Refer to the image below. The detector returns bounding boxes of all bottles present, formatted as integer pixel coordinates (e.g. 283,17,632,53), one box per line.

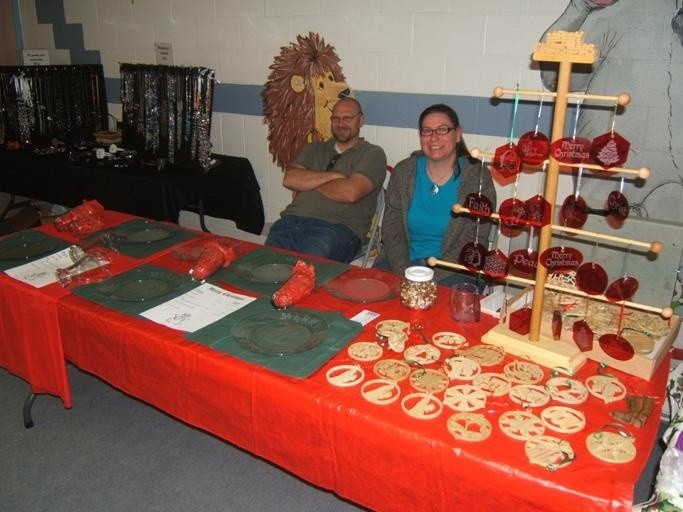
402,266,438,310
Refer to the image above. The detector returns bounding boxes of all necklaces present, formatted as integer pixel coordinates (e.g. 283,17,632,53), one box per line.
428,158,456,195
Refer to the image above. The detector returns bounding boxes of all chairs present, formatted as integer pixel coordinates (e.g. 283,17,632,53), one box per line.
351,164,502,300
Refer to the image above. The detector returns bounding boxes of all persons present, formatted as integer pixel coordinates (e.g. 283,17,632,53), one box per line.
371,104,496,295
264,97,386,264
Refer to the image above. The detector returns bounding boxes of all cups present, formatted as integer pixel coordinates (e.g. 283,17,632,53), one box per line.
54,204,110,288
190,247,226,284
273,272,310,306
451,285,480,320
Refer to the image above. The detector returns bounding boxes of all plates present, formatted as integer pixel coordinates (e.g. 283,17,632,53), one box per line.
105,270,183,302
1,237,55,262
327,270,400,300
234,253,300,285
234,311,329,356
114,222,177,244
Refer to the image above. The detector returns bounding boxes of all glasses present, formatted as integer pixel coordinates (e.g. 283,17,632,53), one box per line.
329,111,359,123
419,125,457,135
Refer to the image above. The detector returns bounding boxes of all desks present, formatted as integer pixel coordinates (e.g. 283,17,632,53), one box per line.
3,148,266,238
1,191,206,431
56,230,682,510
